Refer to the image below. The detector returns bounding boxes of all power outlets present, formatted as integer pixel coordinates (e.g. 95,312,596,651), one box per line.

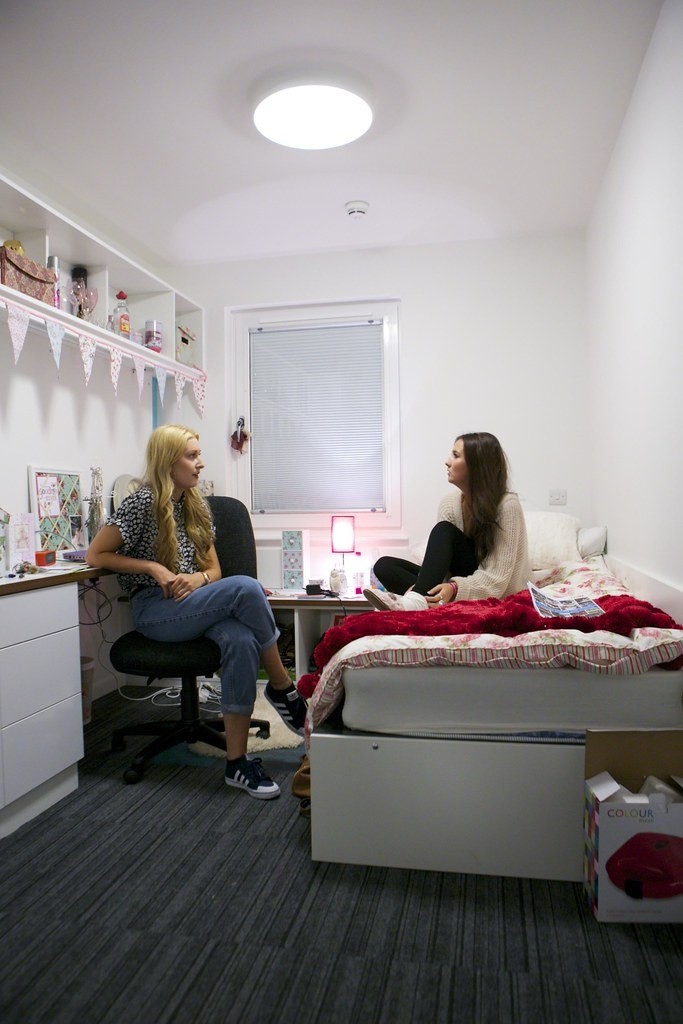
115,593,131,607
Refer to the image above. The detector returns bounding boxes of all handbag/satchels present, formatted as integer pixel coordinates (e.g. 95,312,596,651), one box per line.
291,754,311,815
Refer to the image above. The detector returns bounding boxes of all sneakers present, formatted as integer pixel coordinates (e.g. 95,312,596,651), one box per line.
226,755,281,799
264,679,310,737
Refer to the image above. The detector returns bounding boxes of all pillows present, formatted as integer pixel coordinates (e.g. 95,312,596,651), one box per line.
520,511,583,569
576,524,607,557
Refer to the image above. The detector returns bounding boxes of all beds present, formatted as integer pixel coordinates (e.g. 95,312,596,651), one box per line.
310,553,683,885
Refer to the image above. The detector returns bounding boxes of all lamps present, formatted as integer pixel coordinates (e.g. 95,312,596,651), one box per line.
251,85,376,150
330,515,355,566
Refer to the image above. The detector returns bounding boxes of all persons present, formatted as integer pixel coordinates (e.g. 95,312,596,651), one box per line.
86,424,309,799
362,431,532,612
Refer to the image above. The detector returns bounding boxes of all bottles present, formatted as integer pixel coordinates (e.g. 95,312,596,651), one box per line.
349,551,368,598
113,291,131,340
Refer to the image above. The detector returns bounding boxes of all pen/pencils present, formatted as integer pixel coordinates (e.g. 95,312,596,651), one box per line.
3,573,16,578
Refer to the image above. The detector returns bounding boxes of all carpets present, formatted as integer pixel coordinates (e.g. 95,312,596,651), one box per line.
187,688,304,758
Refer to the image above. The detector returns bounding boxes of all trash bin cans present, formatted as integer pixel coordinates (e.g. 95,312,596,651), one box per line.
81,656,95,725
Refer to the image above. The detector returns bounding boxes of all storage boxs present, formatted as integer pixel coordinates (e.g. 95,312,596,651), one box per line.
581,760,683,925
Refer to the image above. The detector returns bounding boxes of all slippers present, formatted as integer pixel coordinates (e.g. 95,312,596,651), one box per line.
363,588,405,612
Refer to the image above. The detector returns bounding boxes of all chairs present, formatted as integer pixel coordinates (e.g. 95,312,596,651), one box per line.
109,495,271,785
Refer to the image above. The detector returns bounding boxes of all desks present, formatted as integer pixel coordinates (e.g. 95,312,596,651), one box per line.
263,590,376,681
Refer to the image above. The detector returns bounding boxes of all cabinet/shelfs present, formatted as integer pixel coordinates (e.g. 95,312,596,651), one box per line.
0,559,112,841
0,173,205,380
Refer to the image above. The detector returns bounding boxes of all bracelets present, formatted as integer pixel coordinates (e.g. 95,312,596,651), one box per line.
450,582,457,599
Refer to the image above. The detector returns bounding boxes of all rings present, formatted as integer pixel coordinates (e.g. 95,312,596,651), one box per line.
438,594,443,600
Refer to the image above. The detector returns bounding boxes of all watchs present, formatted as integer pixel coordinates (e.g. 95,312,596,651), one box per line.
200,571,211,585
